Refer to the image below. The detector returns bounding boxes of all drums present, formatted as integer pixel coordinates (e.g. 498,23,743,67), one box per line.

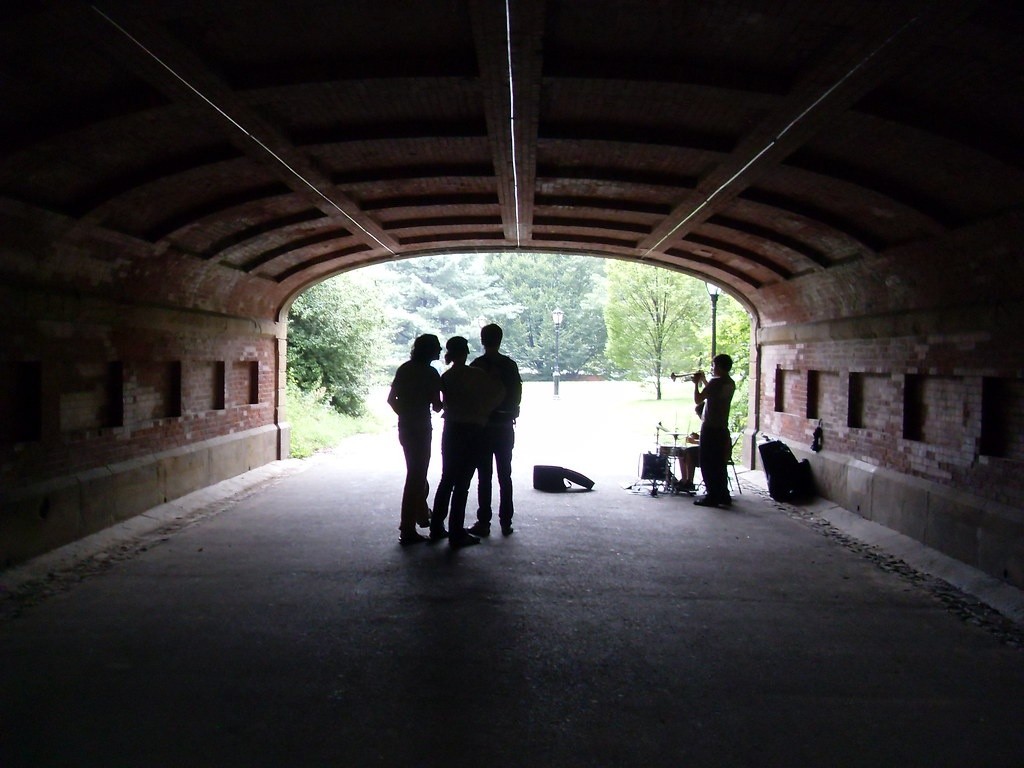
658,445,686,457
639,454,669,480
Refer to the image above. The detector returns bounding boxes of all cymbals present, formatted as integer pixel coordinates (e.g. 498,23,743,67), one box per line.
653,422,670,432
667,433,689,436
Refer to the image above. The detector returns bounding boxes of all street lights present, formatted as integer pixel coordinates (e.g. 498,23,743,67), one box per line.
552,307,564,395
704,280,721,374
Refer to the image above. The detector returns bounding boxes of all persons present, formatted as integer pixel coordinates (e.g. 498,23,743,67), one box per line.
693,354,736,505
387,324,522,548
675,403,732,490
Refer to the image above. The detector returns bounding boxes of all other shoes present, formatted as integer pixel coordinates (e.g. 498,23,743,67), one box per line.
433,528,450,540
674,479,695,490
420,509,433,529
694,498,718,506
502,524,514,536
466,522,490,536
450,533,480,548
399,533,431,546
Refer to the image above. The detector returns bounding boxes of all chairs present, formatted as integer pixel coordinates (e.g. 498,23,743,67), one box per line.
697,432,742,494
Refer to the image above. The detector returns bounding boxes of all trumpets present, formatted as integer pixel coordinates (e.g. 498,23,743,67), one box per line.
671,370,714,388
